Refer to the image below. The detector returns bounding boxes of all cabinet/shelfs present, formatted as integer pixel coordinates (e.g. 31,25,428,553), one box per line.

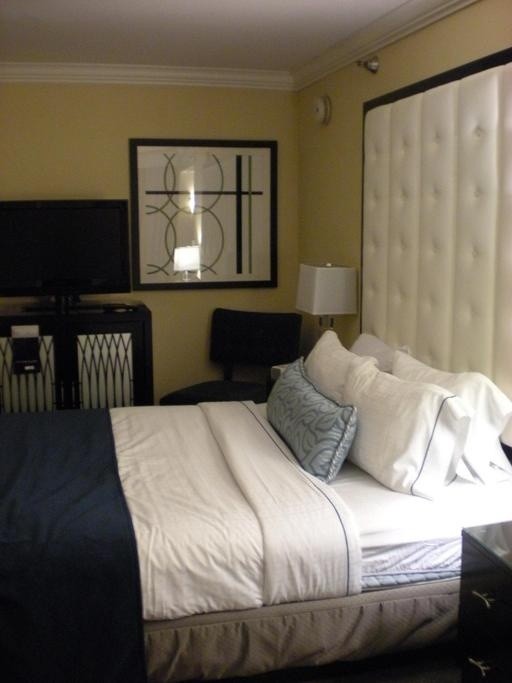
0,300,154,414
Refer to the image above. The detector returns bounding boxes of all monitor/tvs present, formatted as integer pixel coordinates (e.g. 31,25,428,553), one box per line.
0,198,131,312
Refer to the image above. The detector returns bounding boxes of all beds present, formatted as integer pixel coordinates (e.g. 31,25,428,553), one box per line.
0,329,512,683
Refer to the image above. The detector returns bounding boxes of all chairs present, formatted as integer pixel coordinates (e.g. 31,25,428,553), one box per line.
160,307,303,405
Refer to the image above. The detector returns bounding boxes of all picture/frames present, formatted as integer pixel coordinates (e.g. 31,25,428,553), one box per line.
129,139,279,291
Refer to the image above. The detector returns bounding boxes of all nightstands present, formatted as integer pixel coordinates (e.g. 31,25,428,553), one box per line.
459,520,512,683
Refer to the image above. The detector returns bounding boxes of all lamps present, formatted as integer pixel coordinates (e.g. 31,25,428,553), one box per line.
295,261,358,340
172,245,200,281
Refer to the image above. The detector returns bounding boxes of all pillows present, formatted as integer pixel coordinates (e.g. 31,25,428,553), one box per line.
392,350,512,486
266,357,359,483
348,332,409,373
303,328,379,409
340,355,472,501
